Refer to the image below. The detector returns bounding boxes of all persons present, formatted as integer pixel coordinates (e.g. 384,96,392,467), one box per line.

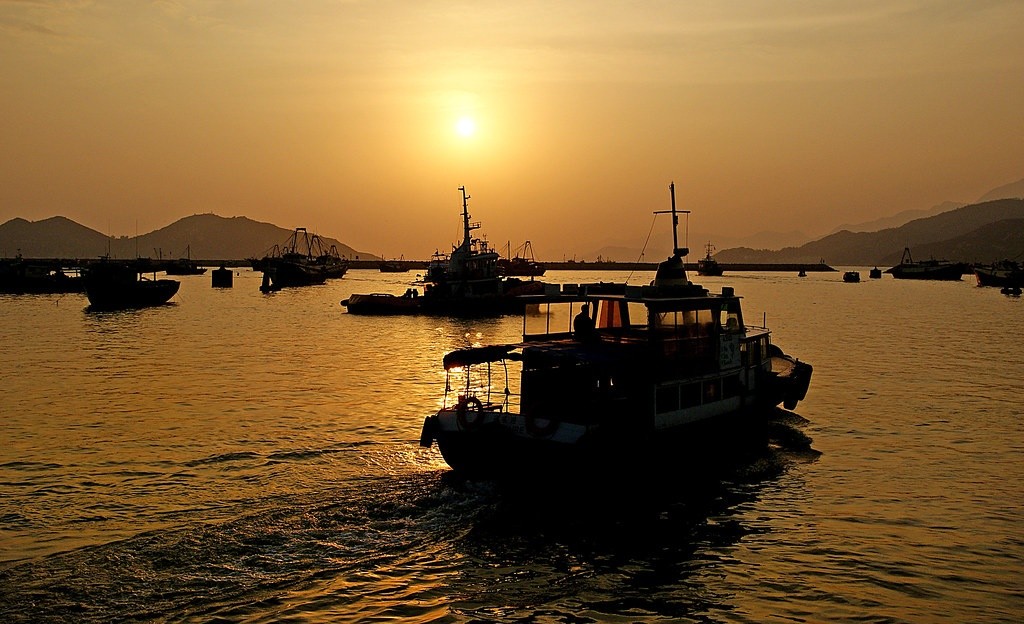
572,303,602,342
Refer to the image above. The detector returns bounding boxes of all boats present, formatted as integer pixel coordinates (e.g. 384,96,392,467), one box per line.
0,252,82,297
696,240,725,276
247,227,351,297
377,254,412,273
798,268,807,277
843,270,860,283
339,186,546,319
83,253,180,314
418,180,814,522
975,260,1024,289
886,246,972,282
138,244,207,275
